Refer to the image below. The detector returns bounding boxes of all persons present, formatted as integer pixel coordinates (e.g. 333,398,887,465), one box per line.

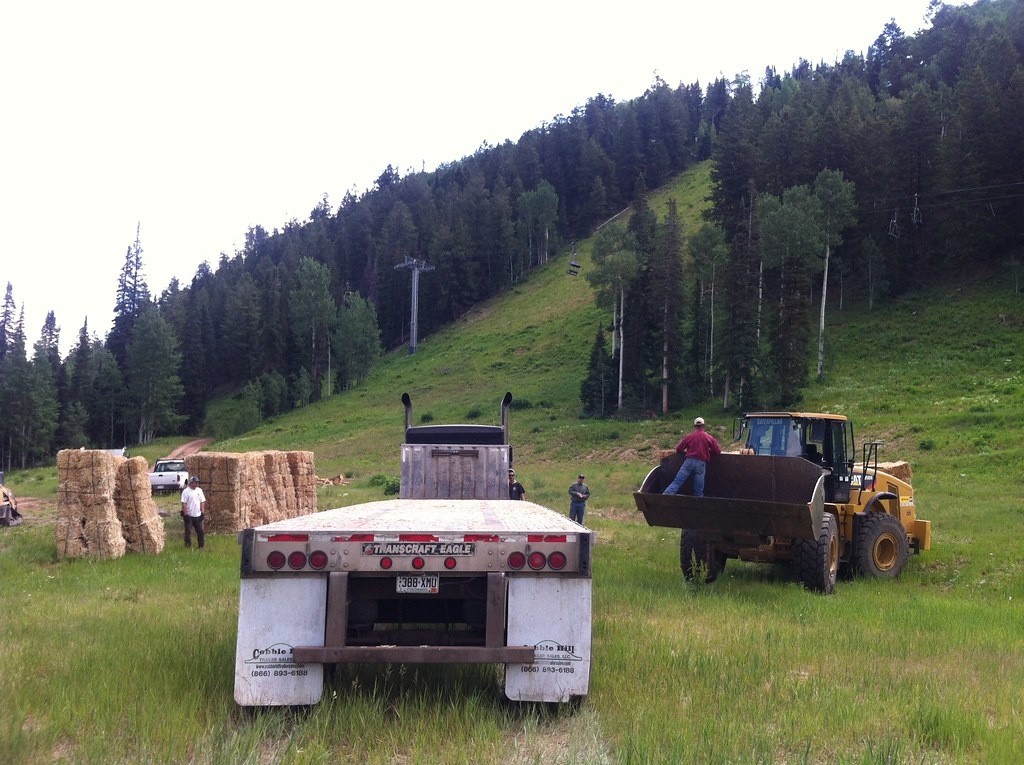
568,474,590,525
663,417,721,497
509,470,526,501
181,477,207,547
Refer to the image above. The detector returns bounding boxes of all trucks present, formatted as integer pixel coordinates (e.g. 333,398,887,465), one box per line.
235,392,597,718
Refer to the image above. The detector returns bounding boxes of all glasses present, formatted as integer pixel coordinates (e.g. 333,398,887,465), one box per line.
509,475,515,477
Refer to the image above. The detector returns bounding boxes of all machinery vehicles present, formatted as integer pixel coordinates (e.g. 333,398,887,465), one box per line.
632,409,931,597
0,471,23,528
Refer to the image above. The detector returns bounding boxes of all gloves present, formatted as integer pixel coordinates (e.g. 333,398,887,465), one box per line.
184,515,188,520
199,513,204,521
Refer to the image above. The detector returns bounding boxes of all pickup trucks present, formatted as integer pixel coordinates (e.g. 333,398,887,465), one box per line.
147,458,190,495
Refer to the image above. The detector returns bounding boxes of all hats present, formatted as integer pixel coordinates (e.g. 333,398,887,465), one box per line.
509,469,516,473
694,417,704,427
190,477,200,483
579,475,584,479
